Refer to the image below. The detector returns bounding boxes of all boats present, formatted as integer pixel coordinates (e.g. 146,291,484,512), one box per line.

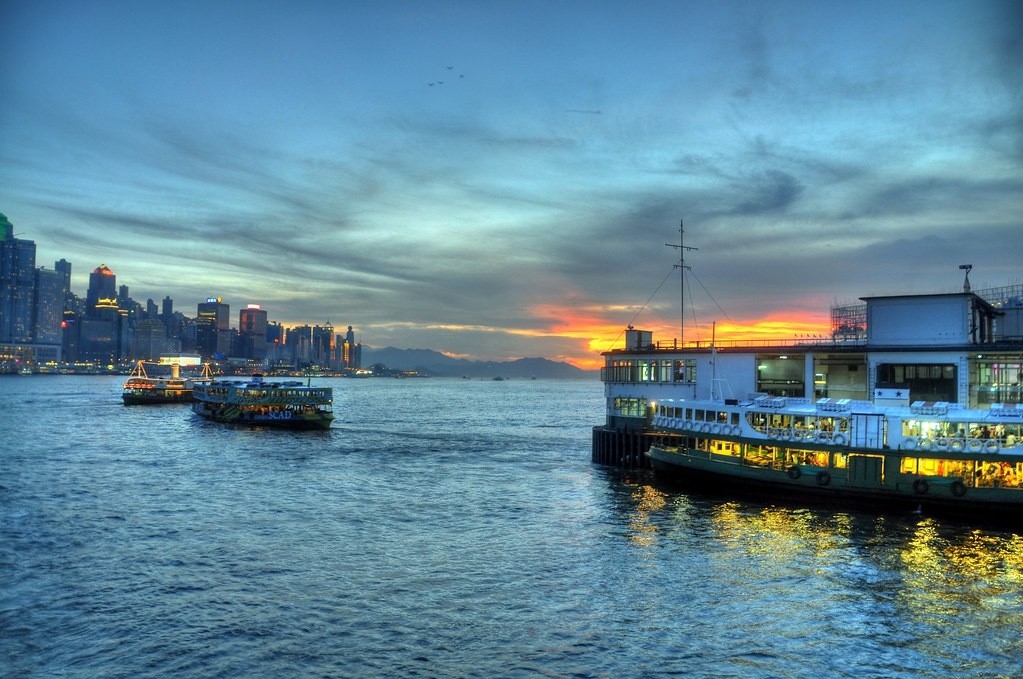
646,320,1023,510
191,362,336,429
121,359,215,405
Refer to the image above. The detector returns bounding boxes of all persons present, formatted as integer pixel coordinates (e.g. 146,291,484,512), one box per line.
980,427,990,439
1007,432,1016,447
795,417,829,437
774,419,790,435
956,429,964,438
961,462,968,476
938,461,943,476
976,462,1016,488
791,451,814,466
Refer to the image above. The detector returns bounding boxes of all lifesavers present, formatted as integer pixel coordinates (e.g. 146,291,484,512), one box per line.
913,479,929,494
950,481,966,497
655,416,742,437
903,437,1000,455
769,427,846,444
816,471,831,485
788,466,801,479
